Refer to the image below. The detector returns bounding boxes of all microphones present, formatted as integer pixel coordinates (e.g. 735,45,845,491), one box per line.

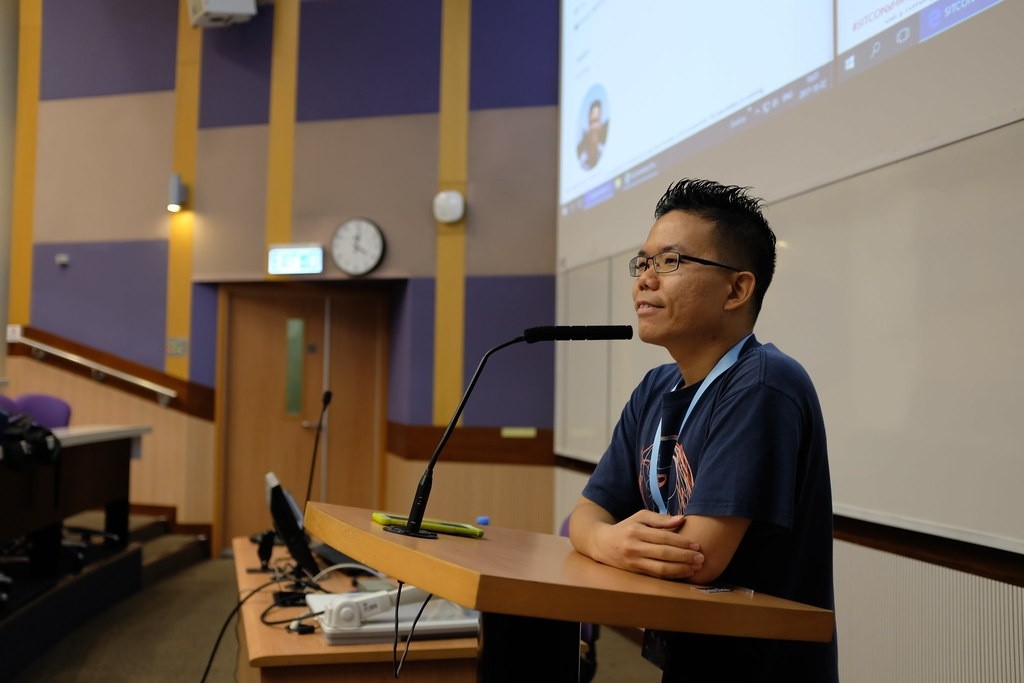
303,390,332,514
405,324,633,532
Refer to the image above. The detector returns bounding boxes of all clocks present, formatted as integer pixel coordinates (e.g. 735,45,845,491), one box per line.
331,218,386,276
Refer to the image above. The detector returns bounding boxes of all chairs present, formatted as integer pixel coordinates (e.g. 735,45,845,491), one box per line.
0,396,72,429
559,512,600,683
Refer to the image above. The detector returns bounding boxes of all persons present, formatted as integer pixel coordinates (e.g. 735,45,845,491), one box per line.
569,179,839,683
578,101,605,171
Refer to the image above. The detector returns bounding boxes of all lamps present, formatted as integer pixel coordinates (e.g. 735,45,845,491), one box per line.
166,172,189,214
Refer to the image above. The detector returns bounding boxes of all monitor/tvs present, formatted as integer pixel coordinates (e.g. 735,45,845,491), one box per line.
266,473,320,576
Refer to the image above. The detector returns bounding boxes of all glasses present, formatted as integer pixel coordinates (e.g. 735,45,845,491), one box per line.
628,251,743,277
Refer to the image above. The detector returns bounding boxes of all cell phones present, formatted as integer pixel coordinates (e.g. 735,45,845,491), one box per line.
371,513,484,537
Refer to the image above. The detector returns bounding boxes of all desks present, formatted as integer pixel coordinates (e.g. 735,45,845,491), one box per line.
0,423,154,611
231,534,477,683
